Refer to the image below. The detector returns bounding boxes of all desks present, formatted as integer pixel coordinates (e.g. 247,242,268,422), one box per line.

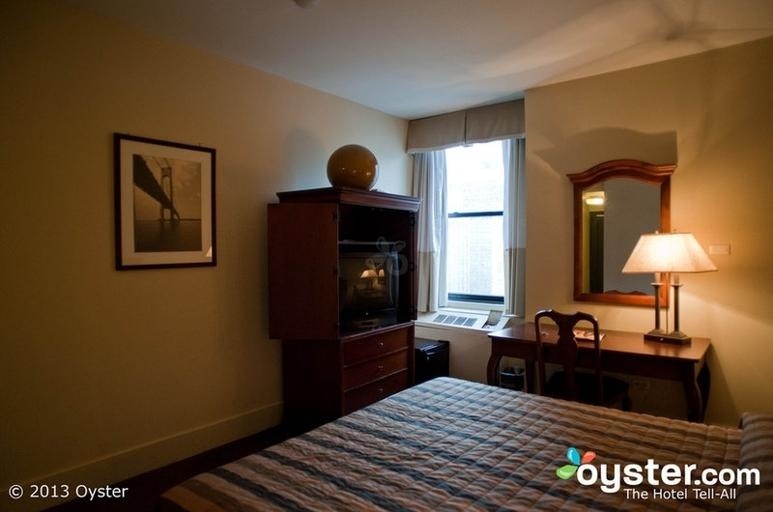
484,320,714,422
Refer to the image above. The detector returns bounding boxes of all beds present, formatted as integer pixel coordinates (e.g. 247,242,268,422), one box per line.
152,376,741,512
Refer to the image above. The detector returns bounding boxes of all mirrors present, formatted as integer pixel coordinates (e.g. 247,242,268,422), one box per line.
563,159,678,308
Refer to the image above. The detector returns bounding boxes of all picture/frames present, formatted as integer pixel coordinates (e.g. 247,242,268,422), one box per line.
113,131,216,274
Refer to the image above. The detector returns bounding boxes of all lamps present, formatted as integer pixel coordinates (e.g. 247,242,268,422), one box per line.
622,230,718,346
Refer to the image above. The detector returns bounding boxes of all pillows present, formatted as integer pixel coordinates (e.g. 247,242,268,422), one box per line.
733,409,771,512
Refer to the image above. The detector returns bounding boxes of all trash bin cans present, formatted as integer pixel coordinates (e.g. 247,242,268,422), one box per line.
498,366,525,392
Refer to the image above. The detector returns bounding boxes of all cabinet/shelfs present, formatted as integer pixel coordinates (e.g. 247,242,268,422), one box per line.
263,187,450,441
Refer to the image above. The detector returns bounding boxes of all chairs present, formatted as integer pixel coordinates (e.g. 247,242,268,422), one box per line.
534,308,604,409
